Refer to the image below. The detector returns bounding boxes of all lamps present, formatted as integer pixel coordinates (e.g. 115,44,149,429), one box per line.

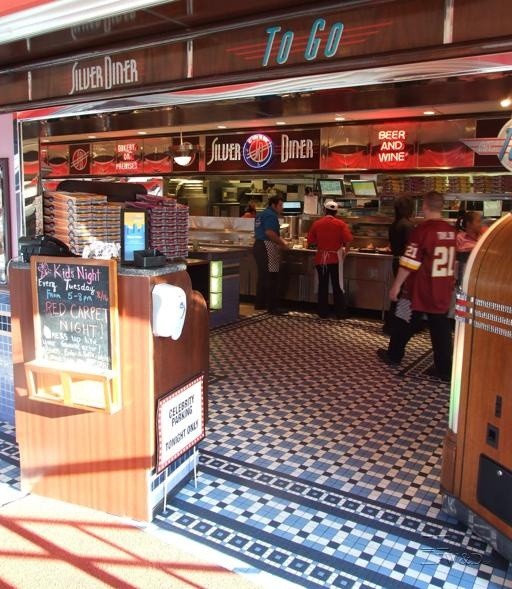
168,132,194,166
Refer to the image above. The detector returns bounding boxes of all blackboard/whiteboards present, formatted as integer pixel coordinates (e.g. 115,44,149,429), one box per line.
30,255,121,371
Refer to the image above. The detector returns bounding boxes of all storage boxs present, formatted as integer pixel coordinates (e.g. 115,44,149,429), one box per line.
376,172,510,200
41,187,192,265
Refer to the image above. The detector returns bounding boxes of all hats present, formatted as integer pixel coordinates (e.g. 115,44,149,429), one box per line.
323,201,338,211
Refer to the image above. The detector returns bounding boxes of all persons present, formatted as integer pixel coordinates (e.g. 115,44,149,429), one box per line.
383,195,415,335
306,200,353,319
455,209,481,252
377,191,456,380
252,195,289,316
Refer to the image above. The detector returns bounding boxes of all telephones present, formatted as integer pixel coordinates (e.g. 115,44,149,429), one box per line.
19,235,70,263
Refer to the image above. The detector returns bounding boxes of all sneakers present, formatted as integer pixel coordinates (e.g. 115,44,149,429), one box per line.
332,313,353,320
254,303,289,316
376,347,402,366
427,366,451,381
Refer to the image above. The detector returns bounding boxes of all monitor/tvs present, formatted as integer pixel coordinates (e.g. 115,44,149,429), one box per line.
350,180,378,197
483,200,502,218
317,179,345,197
